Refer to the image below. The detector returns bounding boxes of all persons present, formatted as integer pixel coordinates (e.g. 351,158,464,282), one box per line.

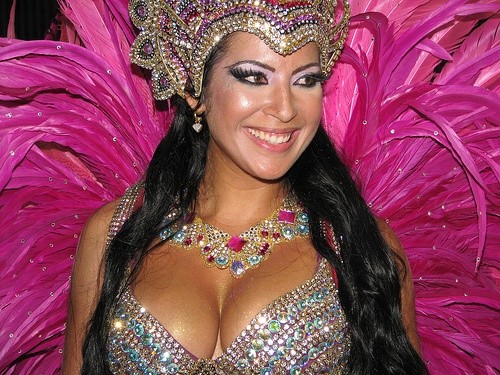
50,0,429,375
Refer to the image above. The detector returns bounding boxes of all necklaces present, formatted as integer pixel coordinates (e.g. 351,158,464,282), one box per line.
157,190,311,279
